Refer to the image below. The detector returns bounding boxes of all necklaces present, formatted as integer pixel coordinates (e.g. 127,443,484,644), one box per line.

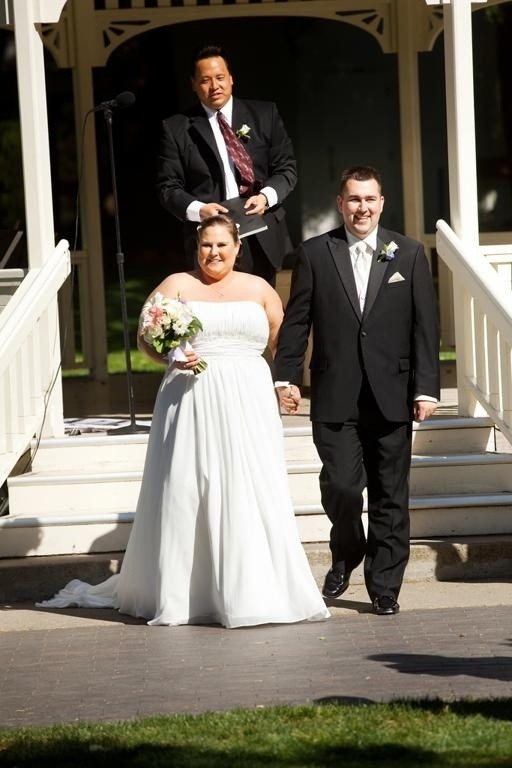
214,285,226,297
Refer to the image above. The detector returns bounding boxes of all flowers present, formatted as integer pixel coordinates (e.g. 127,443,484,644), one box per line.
137,292,212,375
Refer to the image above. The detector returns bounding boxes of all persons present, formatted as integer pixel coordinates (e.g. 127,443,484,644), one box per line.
154,44,300,287
272,160,449,614
132,213,306,633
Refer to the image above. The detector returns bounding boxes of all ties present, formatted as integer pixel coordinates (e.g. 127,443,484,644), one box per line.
351,242,369,316
214,109,256,195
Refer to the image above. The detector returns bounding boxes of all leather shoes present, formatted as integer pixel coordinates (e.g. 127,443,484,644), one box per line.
372,594,400,615
322,564,351,599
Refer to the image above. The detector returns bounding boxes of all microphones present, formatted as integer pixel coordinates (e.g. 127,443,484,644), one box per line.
89,91,136,112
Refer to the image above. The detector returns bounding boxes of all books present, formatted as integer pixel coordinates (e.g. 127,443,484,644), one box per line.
219,195,268,240
